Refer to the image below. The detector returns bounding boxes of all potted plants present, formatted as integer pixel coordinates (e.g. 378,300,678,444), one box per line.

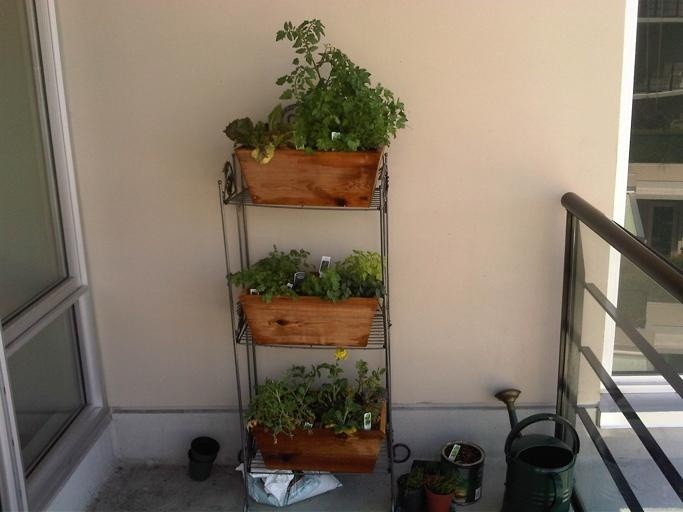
229,17,406,206
236,249,388,347
399,465,430,512
425,469,462,512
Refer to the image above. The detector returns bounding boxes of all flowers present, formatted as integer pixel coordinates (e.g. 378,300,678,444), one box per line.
248,351,384,434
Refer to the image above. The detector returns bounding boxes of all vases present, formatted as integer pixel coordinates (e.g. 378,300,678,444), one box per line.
188,437,220,480
253,425,384,472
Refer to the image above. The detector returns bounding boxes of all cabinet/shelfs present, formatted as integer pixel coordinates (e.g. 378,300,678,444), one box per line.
218,149,407,512
